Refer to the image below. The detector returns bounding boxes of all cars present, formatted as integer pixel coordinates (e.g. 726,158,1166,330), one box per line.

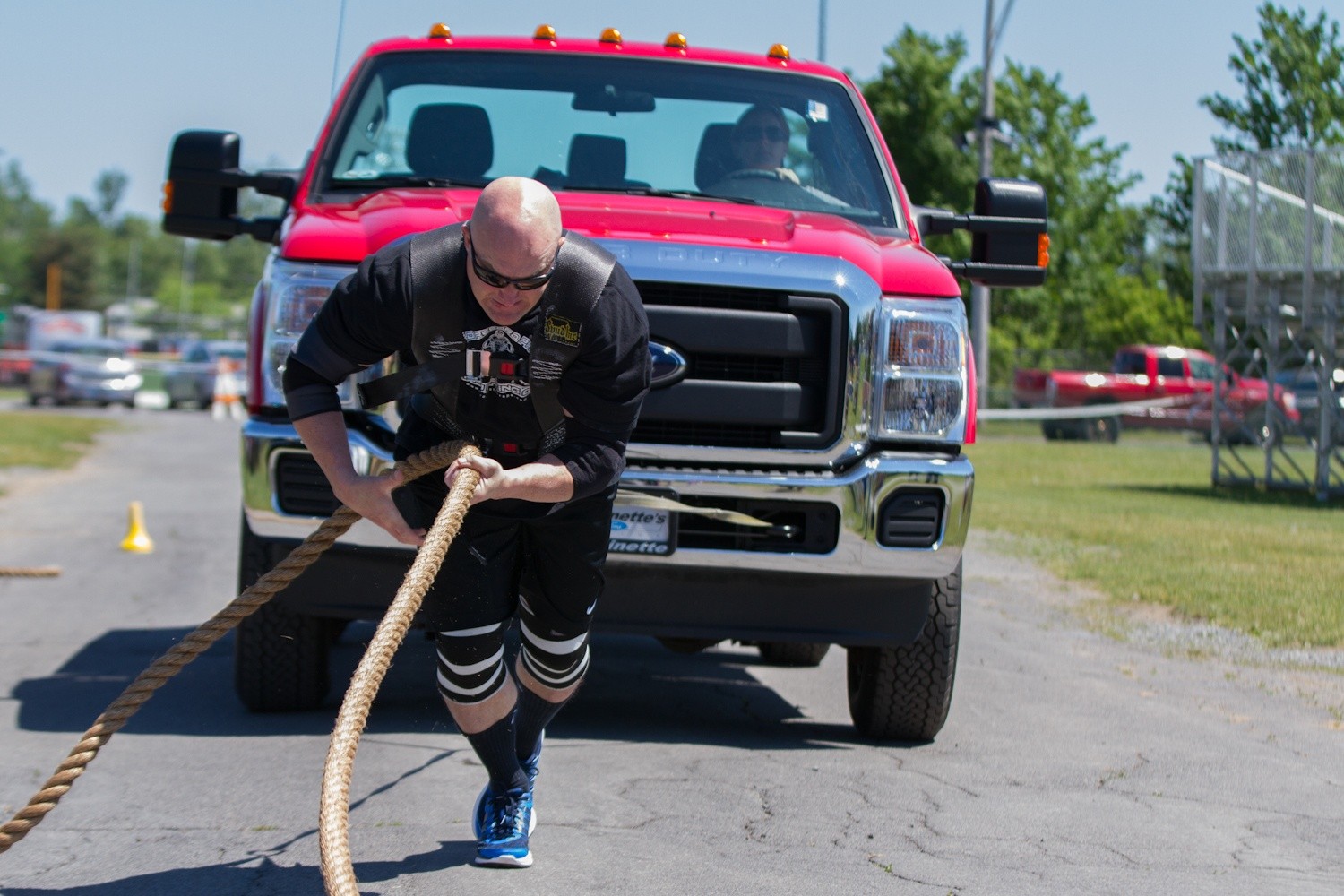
0,305,248,415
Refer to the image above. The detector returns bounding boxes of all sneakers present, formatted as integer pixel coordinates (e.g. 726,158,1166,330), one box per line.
472,720,541,835
475,783,539,868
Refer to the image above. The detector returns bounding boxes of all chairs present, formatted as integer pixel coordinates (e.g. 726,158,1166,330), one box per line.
378,104,496,182
693,123,741,191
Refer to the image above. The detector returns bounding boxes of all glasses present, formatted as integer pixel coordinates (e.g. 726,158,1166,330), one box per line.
743,125,785,142
469,226,559,291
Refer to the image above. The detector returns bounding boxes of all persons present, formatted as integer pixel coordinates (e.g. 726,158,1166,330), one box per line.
725,95,853,207
283,175,652,869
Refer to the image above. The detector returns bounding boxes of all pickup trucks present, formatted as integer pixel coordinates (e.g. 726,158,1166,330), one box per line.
1014,347,1302,447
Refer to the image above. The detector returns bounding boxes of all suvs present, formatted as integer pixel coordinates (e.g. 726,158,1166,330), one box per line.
157,21,1054,753
